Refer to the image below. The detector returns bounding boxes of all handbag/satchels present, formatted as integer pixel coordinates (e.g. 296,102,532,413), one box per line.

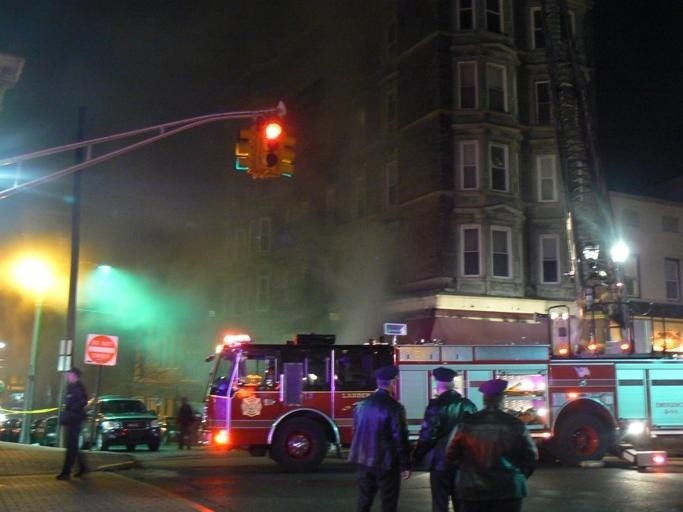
409,444,439,471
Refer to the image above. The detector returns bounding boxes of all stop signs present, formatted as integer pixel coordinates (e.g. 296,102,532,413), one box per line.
83,332,119,367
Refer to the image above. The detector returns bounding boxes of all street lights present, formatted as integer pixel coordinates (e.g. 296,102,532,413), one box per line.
15,254,114,446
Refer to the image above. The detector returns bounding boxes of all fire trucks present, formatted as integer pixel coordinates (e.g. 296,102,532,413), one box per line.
195,1,681,475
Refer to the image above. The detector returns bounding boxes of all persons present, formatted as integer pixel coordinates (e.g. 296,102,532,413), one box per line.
176,396,192,450
56,368,87,480
349,365,413,512
413,367,478,511
444,378,540,512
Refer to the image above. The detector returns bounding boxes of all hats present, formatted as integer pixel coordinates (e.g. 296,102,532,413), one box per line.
479,379,507,395
375,364,398,380
67,367,82,375
433,366,457,382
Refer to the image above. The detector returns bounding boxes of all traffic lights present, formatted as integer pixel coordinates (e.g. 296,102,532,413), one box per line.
273,134,300,178
229,124,257,174
255,115,286,171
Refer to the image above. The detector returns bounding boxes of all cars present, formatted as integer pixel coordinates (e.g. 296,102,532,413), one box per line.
156,412,210,448
0,415,59,448
77,394,162,453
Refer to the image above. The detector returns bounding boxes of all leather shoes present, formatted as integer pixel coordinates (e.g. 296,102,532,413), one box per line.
57,472,69,480
